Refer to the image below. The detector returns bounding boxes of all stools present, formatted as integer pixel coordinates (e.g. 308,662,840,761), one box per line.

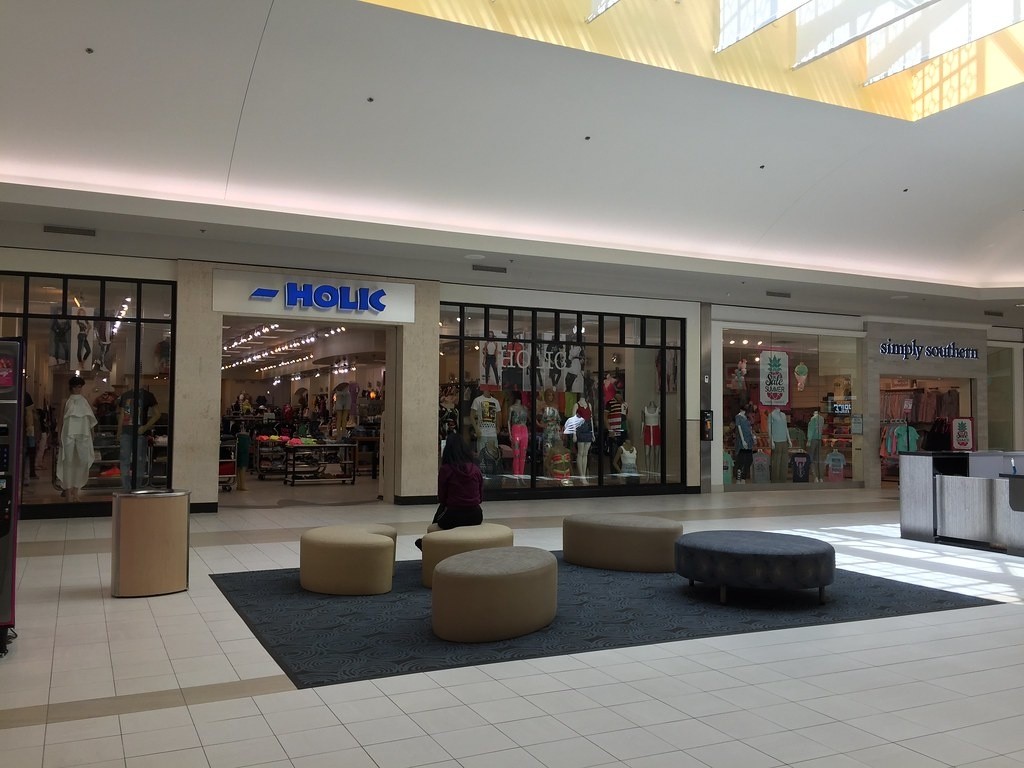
300,523,397,594
674,530,835,605
562,513,683,572
420,522,513,587
430,546,557,641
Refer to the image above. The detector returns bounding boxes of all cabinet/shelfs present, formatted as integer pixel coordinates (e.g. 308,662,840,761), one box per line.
218,413,380,492
51,426,168,490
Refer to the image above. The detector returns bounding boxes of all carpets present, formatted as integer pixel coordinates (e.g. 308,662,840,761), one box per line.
209,551,1006,691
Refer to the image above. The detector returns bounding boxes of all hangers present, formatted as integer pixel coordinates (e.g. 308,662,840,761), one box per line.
882,419,909,425
884,387,958,396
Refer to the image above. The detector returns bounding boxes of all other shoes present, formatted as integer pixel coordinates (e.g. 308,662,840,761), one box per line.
99,364,110,372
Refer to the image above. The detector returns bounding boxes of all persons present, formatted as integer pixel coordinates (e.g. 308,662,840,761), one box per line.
602,373,617,412
546,334,562,392
237,431,249,490
116,374,160,495
805,411,825,483
94,313,114,373
470,390,503,454
415,428,483,568
767,408,793,484
505,400,528,475
481,331,500,389
440,399,458,439
734,409,757,484
56,376,98,503
54,307,91,367
571,396,595,486
536,388,564,476
604,394,628,479
476,440,503,473
503,334,523,391
528,336,544,389
334,388,351,442
613,439,638,481
154,335,170,374
565,337,584,394
640,402,662,483
